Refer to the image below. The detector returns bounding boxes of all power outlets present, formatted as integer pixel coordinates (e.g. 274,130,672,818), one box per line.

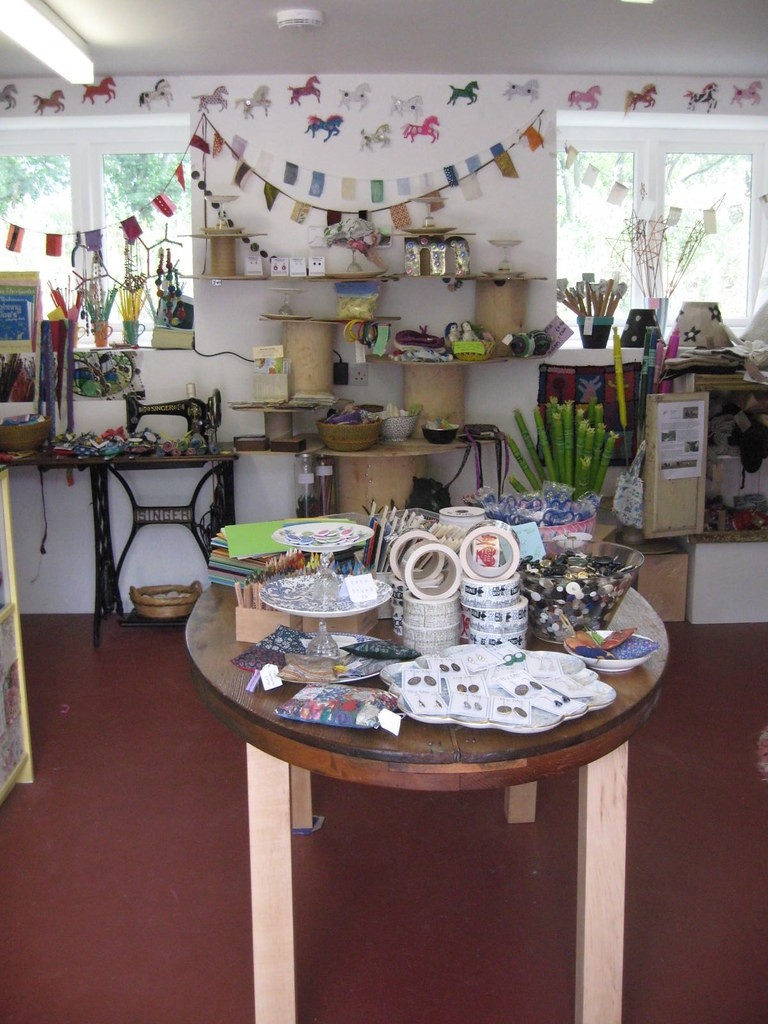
350,364,368,386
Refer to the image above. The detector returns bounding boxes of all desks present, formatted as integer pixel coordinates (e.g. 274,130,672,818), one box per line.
185,581,667,1024
314,441,494,516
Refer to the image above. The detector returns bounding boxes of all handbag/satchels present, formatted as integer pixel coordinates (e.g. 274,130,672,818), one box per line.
610,439,645,529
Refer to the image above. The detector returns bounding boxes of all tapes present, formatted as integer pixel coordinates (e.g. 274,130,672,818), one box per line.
461,520,520,582
401,620,462,655
467,624,527,650
468,595,530,634
389,572,445,608
392,606,403,636
402,589,462,627
460,571,521,609
400,540,462,601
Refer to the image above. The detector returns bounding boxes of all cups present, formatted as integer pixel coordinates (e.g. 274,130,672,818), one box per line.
94,321,113,347
123,320,145,345
74,322,86,347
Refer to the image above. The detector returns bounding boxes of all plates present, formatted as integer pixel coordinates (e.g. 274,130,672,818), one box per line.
258,575,393,617
323,272,386,279
261,314,313,320
271,523,374,552
482,271,527,277
268,631,396,683
489,240,523,246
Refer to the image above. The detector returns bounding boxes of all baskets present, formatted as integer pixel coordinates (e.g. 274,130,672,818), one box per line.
130,580,203,619
0,414,52,451
317,403,385,450
452,341,498,361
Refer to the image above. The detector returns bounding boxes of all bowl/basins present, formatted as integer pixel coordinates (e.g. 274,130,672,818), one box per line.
381,416,418,442
563,630,654,672
515,539,645,645
314,417,383,451
421,424,460,444
0,414,53,452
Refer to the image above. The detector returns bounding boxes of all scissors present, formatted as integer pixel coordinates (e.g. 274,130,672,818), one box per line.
483,490,600,526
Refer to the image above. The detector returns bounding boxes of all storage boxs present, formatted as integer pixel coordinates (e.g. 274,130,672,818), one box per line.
382,508,439,519
322,512,370,529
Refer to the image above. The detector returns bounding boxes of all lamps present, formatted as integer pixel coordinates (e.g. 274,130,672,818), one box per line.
0,0,94,85
276,10,324,29
